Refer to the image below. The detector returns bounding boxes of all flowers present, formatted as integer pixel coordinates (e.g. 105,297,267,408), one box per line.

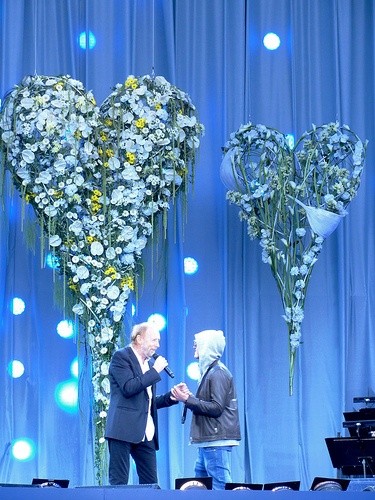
0,75,204,488
222,122,367,396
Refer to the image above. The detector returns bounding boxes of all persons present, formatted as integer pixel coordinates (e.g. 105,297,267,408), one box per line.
169,330,241,491
103,322,192,486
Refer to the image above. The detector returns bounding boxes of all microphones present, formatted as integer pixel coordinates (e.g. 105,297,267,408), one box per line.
152,353,175,378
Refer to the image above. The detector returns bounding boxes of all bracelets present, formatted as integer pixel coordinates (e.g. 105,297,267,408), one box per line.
184,394,191,404
